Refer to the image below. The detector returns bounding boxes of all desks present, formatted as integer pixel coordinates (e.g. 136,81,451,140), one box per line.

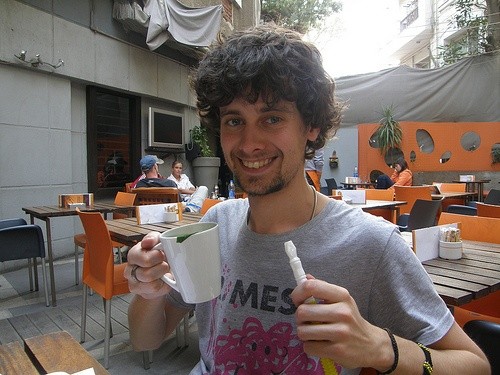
340,183,372,190
347,200,408,225
104,213,203,363
431,192,478,206
453,180,492,206
399,232,500,306
22,203,137,307
0,330,112,375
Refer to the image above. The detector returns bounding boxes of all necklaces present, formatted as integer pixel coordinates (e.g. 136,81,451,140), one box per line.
247,185,317,226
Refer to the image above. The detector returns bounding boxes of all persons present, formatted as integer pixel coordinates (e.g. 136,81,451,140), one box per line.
305,146,324,192
167,159,195,197
386,157,412,192
123,12,492,375
134,154,182,206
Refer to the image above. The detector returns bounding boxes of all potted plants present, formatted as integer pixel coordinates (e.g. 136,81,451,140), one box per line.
189,126,221,196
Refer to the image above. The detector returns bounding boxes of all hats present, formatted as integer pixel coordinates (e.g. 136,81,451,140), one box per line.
140,154,164,171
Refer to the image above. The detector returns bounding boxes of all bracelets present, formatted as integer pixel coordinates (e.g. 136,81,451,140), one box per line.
376,328,398,375
416,342,433,375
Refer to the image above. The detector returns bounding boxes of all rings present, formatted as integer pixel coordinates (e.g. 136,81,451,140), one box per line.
130,265,140,283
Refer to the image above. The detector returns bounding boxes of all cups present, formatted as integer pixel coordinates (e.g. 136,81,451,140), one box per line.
162,212,179,223
439,241,462,259
149,222,222,304
344,199,353,205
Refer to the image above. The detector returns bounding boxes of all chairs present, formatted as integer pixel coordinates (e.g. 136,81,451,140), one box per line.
0,183,500,375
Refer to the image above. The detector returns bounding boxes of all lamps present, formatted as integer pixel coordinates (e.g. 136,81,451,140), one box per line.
14,50,64,69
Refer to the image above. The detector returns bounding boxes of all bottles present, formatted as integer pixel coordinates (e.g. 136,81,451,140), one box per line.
214,184,218,198
210,192,215,199
228,180,235,199
354,167,359,177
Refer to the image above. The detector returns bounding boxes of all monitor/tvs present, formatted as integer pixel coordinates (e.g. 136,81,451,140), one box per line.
148,107,186,149
